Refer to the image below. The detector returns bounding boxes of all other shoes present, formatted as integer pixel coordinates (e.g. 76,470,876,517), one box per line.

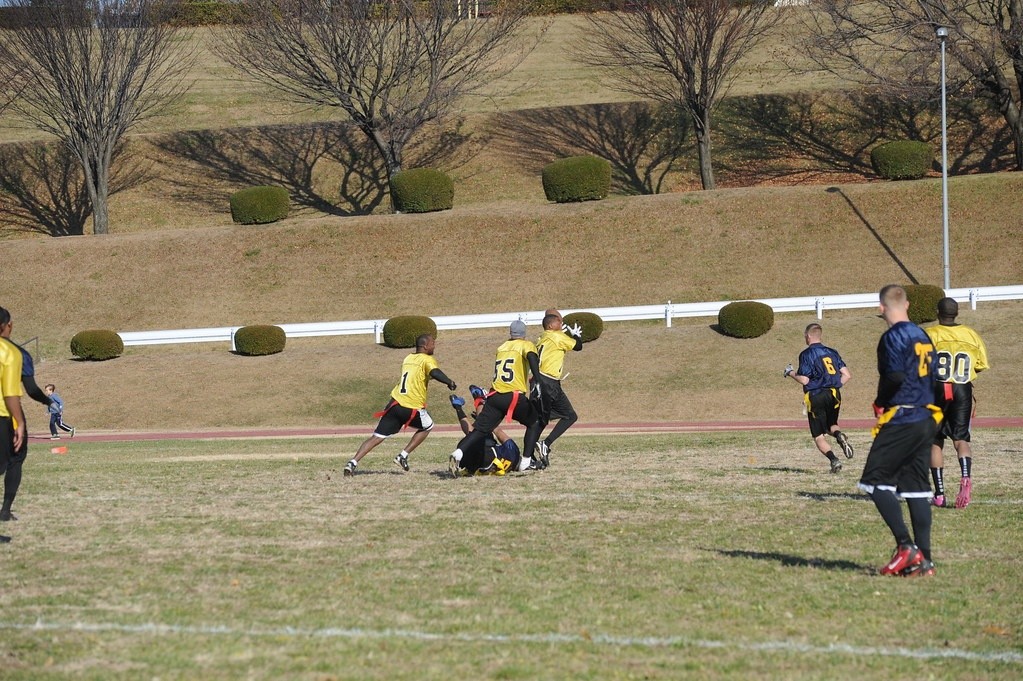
50,434,60,440
0,510,17,521
70,428,75,437
0,535,10,543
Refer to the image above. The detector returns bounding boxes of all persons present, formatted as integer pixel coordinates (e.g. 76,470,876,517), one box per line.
923,297,990,508
450,320,542,479
531,315,582,466
449,384,522,477
0,306,25,543
344,334,457,474
45,384,75,440
857,284,947,576
784,323,853,473
0,309,61,520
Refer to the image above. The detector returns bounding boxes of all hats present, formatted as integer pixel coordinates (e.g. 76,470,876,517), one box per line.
509,321,526,338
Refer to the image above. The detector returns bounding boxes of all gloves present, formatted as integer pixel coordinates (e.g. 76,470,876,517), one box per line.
447,382,457,390
530,383,541,399
561,322,567,332
783,363,794,377
567,323,582,337
50,403,61,413
471,411,476,419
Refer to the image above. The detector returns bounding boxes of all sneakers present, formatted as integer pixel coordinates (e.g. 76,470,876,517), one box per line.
469,385,487,401
894,560,936,577
535,440,551,466
448,454,460,479
955,477,971,509
831,459,842,474
523,459,546,471
880,544,926,576
343,461,356,477
837,432,853,459
930,494,946,508
393,454,409,471
449,394,465,406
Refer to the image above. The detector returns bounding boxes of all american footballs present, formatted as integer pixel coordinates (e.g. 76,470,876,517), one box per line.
545,309,563,324
